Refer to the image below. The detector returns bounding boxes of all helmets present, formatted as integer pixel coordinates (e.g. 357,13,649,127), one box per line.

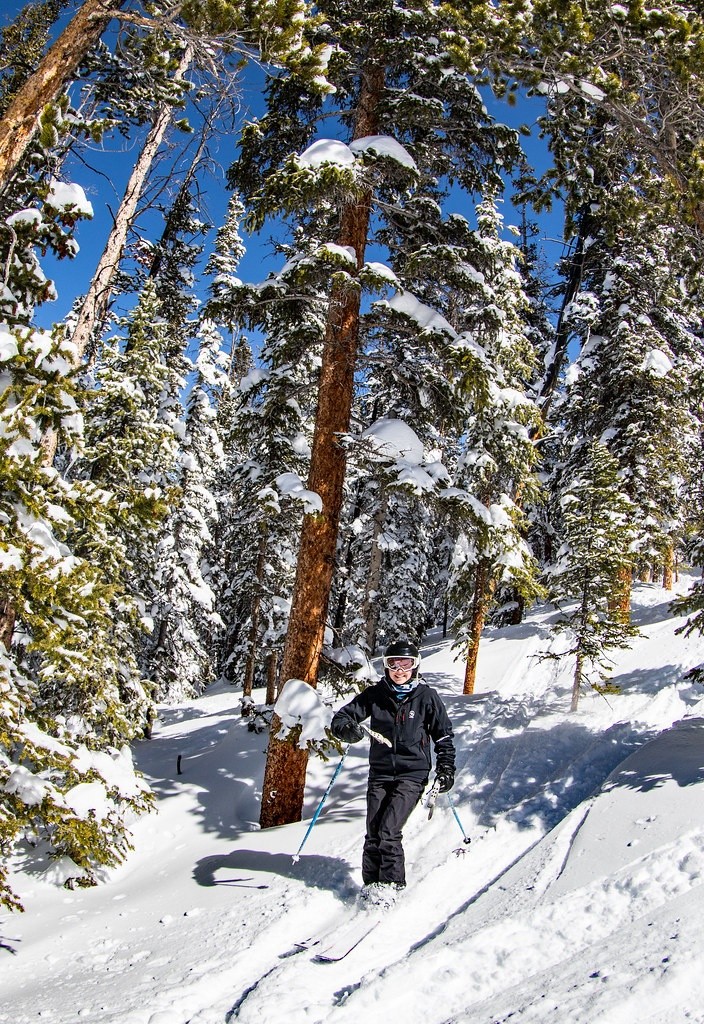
383,641,419,660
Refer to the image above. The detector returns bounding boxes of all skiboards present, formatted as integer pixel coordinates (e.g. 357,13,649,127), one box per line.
292,856,436,962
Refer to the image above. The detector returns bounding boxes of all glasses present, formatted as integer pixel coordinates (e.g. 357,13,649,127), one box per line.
383,655,418,671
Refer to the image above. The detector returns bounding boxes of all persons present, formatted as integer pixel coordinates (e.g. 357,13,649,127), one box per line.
330,641,456,910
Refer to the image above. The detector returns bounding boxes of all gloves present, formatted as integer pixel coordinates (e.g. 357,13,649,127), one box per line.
432,773,454,793
342,722,364,744
